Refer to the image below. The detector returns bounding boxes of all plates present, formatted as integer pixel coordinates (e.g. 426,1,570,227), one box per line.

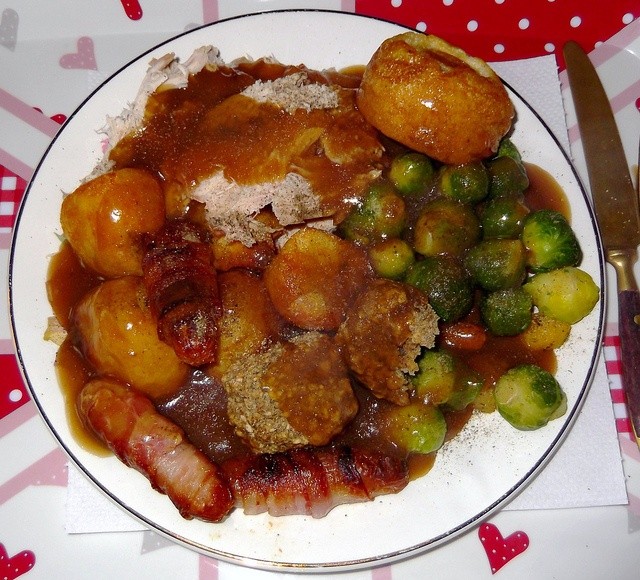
7,6,606,572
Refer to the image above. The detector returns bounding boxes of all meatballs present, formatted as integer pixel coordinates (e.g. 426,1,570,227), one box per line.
62,29,516,456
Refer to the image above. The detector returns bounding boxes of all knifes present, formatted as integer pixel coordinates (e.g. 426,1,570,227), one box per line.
561,39,640,454
